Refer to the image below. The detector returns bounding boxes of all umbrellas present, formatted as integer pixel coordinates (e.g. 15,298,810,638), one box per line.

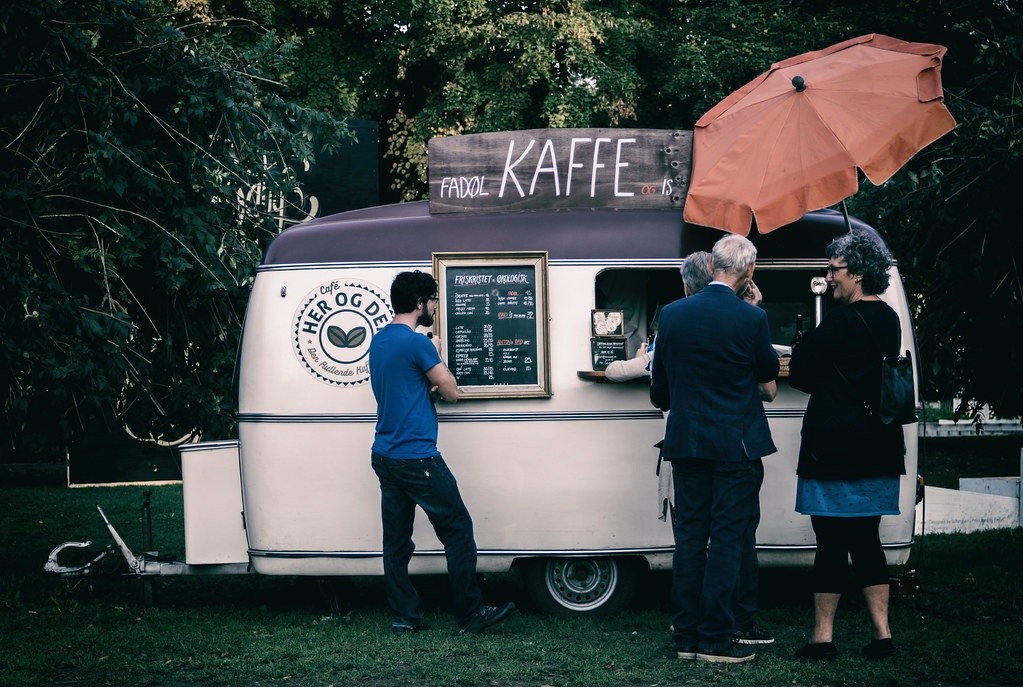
682,30,959,236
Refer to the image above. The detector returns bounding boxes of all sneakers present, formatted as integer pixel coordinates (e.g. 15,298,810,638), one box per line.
392,619,430,633
466,602,515,633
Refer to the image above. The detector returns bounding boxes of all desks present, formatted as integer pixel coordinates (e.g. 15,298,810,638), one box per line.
69,477,184,559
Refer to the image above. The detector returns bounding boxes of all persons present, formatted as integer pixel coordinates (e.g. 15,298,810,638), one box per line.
602,233,779,666
369,271,514,633
791,248,904,663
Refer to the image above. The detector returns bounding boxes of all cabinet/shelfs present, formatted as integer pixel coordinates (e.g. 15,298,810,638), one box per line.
179,439,249,567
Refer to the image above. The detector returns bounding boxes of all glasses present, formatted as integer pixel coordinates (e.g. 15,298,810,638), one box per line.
827,265,848,277
428,296,439,303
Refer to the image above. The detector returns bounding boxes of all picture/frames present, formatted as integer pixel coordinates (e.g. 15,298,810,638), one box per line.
430,251,555,402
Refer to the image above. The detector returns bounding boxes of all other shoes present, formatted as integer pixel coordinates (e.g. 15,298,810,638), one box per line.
862,638,895,657
796,642,836,659
675,640,757,664
733,625,776,643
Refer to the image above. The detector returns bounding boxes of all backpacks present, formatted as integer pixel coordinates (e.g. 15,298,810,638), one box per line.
832,304,919,424
